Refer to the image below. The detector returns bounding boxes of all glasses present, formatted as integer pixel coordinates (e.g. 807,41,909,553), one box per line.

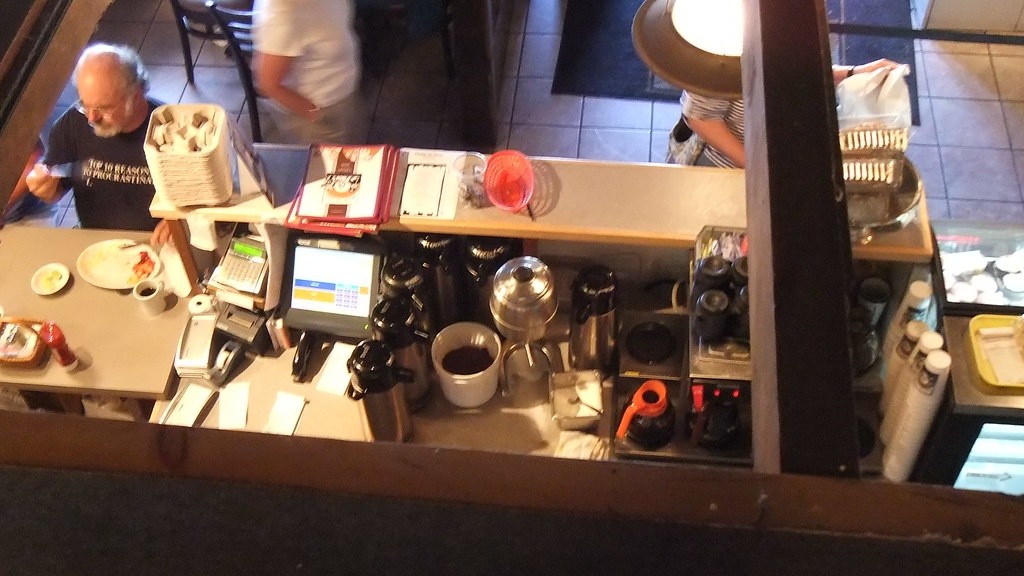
73,96,130,119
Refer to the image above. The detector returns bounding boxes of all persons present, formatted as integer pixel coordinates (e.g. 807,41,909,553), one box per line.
254,0,359,145
26,46,173,244
665,57,903,168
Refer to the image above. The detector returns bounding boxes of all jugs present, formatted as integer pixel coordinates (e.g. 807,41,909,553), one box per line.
344,338,414,442
379,255,439,355
413,233,461,327
565,265,616,372
683,385,745,453
493,254,558,342
615,378,677,452
462,235,510,321
370,297,433,411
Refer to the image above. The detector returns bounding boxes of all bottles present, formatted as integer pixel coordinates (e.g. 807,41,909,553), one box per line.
42,320,78,373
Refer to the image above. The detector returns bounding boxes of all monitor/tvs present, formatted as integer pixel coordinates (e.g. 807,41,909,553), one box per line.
279,237,385,340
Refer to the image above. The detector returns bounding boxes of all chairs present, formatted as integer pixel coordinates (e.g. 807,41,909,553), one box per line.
170,0,253,86
205,1,272,143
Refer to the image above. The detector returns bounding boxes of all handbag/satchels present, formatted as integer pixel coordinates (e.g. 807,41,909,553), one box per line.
668,115,706,166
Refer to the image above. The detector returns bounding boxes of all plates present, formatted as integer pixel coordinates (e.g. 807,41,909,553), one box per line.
77,239,161,290
31,262,70,295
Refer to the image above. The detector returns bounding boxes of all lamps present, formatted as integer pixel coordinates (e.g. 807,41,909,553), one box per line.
630,0,743,101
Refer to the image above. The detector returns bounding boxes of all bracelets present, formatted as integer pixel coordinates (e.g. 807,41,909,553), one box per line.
847,65,856,77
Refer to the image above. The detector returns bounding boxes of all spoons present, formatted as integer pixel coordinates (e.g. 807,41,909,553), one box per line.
568,395,607,417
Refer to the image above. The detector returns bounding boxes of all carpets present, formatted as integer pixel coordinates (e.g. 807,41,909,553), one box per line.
548,0,921,127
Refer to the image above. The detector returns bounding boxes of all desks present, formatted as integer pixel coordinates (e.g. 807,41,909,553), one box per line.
0,224,191,422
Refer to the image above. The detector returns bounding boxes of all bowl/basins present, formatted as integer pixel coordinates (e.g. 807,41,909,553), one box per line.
549,369,604,430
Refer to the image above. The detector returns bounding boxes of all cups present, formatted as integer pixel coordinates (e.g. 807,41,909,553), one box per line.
431,322,502,408
452,150,488,209
856,275,951,484
687,252,749,345
135,278,167,317
